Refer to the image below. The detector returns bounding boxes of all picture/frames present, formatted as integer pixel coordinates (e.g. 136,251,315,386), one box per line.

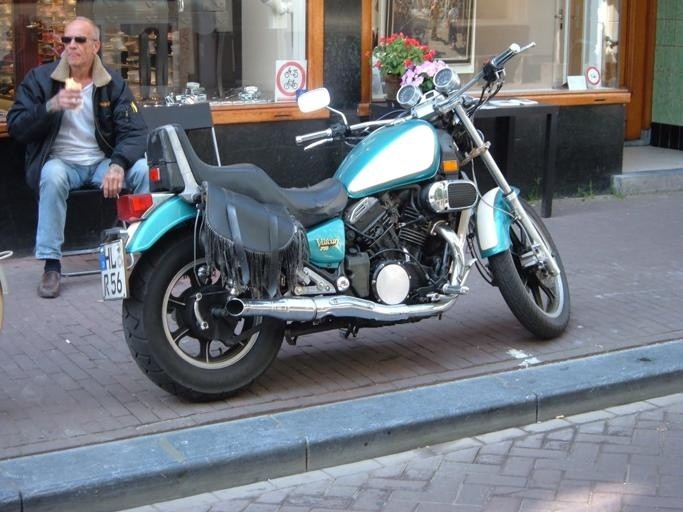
388,1,478,75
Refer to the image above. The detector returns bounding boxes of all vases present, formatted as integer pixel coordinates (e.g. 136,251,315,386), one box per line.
379,75,401,103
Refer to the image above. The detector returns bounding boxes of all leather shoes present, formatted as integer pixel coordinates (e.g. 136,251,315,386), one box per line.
36,267,62,298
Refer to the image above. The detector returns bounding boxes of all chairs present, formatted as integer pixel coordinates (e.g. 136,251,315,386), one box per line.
42,103,224,280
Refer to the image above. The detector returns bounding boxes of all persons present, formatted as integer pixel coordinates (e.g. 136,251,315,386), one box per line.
7,15,152,298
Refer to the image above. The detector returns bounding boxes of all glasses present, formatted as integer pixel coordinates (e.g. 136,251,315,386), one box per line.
59,35,98,44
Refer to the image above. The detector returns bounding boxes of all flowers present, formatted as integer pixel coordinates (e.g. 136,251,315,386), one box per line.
363,33,438,78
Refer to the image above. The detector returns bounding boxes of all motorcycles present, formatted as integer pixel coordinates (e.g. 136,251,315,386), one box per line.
96,42,570,402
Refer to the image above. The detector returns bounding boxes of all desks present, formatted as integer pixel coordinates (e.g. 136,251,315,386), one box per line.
365,94,561,219
